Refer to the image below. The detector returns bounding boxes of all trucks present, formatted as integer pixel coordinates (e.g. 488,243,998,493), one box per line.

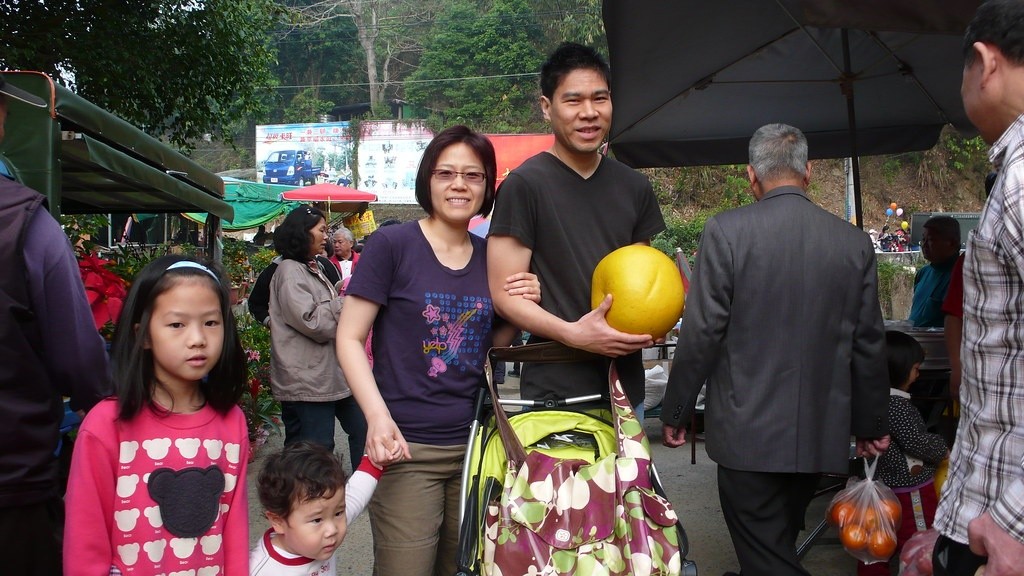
262,150,321,187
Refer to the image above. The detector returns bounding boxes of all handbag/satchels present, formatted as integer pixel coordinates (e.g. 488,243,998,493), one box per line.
825,451,903,566
898,527,941,576
479,337,682,576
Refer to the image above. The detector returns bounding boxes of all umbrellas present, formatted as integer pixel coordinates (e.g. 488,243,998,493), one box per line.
278,182,378,221
603,0,980,231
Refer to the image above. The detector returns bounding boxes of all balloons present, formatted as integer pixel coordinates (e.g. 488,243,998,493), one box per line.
887,203,908,231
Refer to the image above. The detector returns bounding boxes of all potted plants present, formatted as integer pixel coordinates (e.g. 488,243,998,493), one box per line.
222,234,252,304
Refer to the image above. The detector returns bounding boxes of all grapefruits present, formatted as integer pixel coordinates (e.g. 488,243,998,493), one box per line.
591,244,683,340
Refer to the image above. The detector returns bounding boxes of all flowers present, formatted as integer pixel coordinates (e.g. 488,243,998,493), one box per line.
234,338,281,441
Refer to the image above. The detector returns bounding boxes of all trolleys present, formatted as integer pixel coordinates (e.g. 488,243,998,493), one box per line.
643,343,707,433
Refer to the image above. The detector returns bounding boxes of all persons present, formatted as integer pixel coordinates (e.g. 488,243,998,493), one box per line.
660,122,891,576
0,104,117,576
931,0,1024,576
253,225,266,246
249,440,405,576
857,331,950,576
63,253,250,576
906,172,999,450
487,41,685,432
336,126,542,576
879,226,889,238
494,332,524,384
248,204,405,474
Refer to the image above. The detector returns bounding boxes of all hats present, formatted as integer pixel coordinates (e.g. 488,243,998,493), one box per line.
0,72,47,108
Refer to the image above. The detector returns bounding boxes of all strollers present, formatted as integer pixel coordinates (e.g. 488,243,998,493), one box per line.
458,341,698,576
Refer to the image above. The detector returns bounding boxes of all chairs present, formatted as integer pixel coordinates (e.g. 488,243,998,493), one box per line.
796,368,958,558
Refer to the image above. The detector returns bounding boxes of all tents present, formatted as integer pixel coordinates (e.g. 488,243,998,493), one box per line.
132,176,313,255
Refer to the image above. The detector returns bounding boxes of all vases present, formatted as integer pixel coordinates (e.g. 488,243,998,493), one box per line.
248,441,256,463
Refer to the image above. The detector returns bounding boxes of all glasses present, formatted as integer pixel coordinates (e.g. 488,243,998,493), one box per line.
431,169,488,184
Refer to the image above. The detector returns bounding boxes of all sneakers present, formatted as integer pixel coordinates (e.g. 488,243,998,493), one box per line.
508,371,520,377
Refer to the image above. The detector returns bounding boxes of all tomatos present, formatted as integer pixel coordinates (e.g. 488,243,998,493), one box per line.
831,499,901,557
